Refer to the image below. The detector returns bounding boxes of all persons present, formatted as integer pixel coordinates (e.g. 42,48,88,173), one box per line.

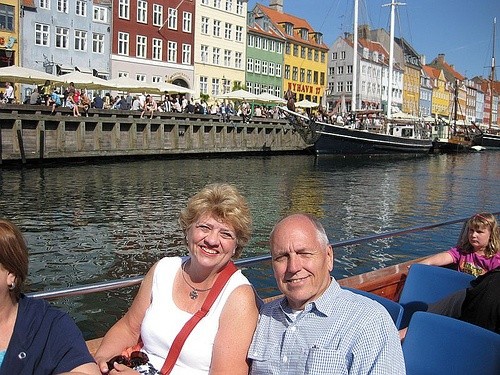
247,214,406,375
0,220,102,375
396,266,500,344
3,81,15,104
94,183,264,375
407,212,500,278
28,79,386,133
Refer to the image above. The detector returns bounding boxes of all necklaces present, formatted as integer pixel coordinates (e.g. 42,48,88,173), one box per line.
182,259,212,300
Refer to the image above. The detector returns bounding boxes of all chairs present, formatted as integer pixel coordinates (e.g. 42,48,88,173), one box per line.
249,264,500,375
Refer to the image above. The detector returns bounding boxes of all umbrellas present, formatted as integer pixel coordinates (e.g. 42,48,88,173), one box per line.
58,71,118,97
153,81,194,103
259,92,287,102
0,65,64,99
215,89,269,102
295,99,318,108
107,74,160,98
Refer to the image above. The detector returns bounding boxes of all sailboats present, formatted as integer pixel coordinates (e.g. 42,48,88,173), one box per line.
278,0,433,154
433,16,500,150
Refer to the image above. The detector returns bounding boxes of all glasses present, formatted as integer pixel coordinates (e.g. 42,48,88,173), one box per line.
106,351,149,372
